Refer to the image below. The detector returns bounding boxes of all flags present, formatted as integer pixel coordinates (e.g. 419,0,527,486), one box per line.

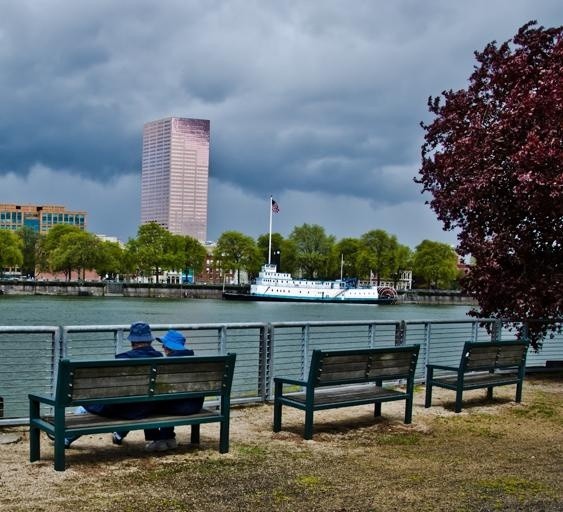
272,199,280,214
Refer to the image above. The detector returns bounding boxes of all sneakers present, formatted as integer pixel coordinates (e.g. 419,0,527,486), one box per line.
166,438,178,448
113,432,123,444
46,432,69,448
145,440,168,451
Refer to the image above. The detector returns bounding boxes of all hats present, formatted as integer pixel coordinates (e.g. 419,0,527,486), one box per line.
155,329,190,351
126,321,154,342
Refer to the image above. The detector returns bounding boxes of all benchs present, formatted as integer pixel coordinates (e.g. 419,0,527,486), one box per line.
28,352,237,472
274,343,421,440
425,339,531,414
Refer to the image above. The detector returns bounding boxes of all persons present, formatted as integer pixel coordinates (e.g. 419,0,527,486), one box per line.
27,273,31,279
46,321,164,448
143,330,204,452
105,272,109,279
32,276,35,280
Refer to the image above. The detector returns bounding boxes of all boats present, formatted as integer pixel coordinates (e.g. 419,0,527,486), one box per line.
220,194,402,303
